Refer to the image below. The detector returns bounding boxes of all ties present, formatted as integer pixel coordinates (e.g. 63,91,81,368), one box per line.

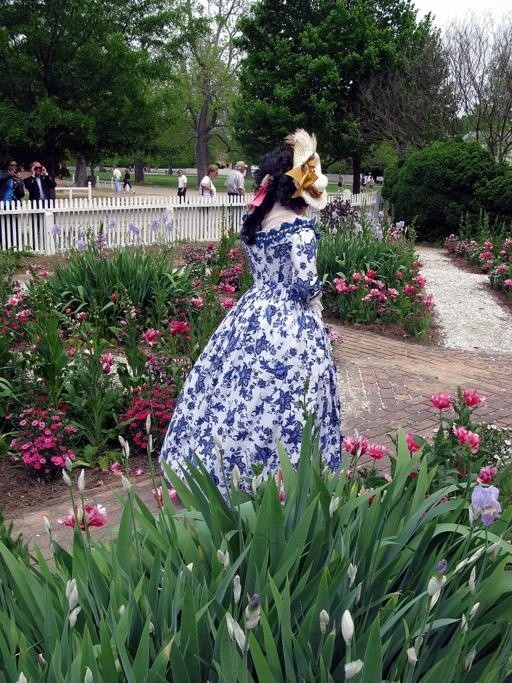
35,177,45,201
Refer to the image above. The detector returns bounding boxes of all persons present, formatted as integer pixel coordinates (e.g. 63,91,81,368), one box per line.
360,171,374,188
0,160,57,250
338,173,343,189
112,165,135,195
158,143,341,508
177,161,248,232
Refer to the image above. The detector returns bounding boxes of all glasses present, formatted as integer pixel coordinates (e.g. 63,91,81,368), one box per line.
34,167,42,171
10,164,18,167
215,172,218,175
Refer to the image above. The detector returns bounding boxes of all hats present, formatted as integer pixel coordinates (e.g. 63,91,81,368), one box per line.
235,161,248,168
176,170,183,174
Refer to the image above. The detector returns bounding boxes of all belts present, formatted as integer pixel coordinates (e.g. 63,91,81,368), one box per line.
228,193,239,196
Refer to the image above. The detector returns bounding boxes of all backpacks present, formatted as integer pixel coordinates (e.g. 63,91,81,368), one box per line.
13,177,25,200
